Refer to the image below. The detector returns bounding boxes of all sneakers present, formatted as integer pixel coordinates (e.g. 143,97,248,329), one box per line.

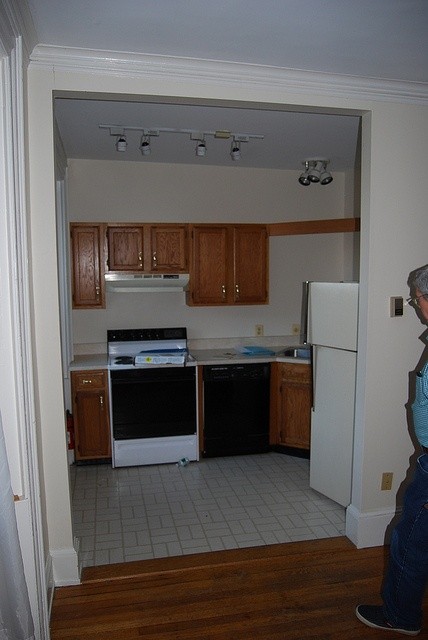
355,604,421,636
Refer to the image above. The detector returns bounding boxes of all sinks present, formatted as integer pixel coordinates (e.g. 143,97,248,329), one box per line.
284,348,312,359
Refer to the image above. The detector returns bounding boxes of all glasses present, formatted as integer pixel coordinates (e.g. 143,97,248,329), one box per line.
406,294,427,306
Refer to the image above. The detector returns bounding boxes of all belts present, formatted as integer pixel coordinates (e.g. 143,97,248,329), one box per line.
419,445,428,455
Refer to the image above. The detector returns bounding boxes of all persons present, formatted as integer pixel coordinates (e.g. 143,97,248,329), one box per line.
354,265,428,633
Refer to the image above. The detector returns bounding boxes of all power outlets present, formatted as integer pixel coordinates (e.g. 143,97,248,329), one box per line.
255,324,264,337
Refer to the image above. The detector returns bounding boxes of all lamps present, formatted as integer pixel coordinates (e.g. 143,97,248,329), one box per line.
141,136,152,155
197,140,206,156
231,138,241,162
298,158,333,186
117,136,127,152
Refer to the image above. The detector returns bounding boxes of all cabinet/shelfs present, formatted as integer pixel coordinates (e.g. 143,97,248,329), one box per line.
71,371,112,462
69,221,106,309
189,224,269,306
272,363,312,456
106,222,190,275
